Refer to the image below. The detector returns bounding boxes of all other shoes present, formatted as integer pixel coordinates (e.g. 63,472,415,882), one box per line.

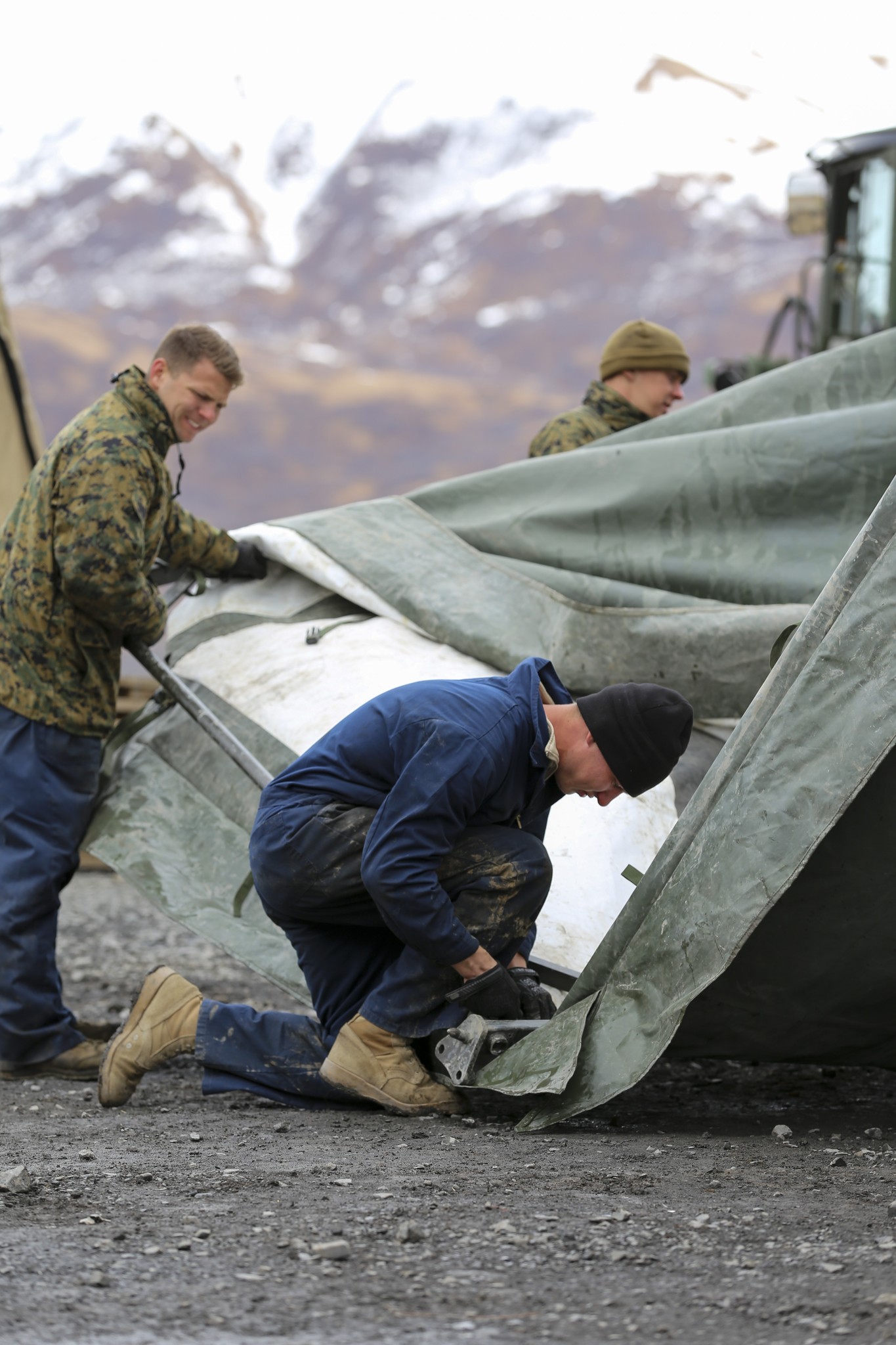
0,1038,108,1080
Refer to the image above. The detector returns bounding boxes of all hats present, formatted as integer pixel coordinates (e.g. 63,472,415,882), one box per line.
576,683,694,798
599,319,690,385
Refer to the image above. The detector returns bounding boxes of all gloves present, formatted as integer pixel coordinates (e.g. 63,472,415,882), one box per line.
218,545,267,583
506,967,557,1020
444,959,524,1020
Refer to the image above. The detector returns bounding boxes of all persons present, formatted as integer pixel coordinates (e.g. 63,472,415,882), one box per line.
530,314,689,460
96,653,697,1115
1,324,268,1080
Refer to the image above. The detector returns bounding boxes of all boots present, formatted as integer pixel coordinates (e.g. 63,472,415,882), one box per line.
318,1013,466,1116
99,964,205,1108
